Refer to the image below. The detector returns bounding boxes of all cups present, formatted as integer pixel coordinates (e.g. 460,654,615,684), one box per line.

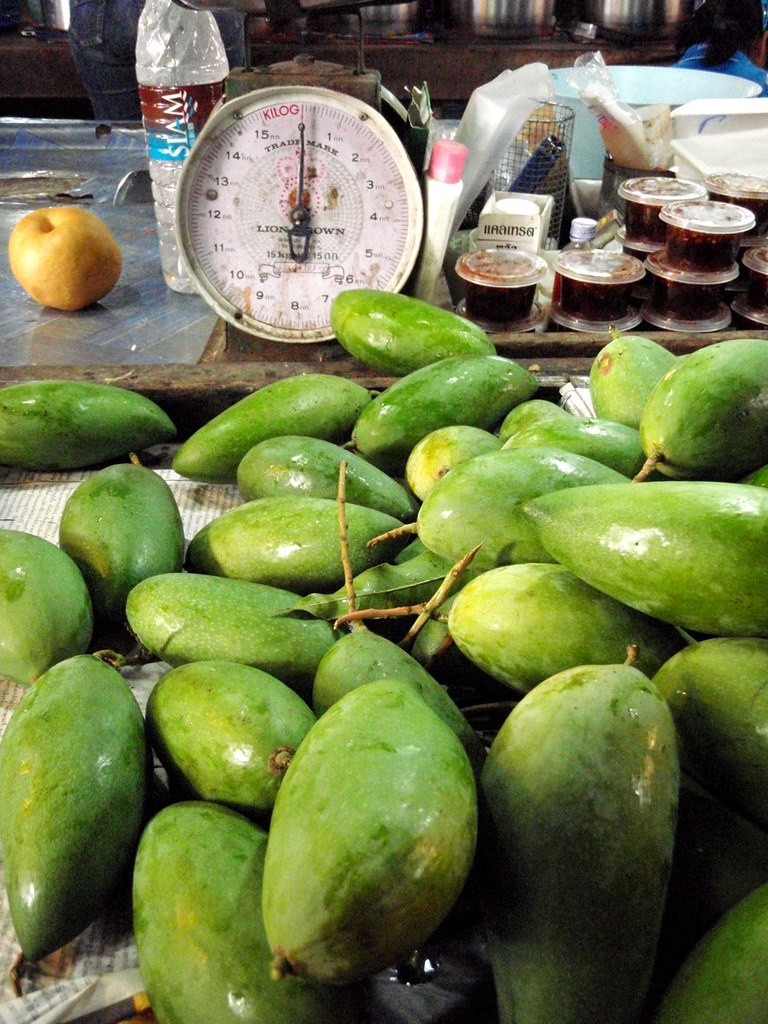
596,155,678,223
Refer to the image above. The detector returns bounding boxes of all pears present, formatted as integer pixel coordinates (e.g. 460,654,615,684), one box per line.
7,206,121,312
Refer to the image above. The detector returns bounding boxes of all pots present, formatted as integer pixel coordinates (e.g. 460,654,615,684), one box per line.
443,0,558,39
589,2,707,41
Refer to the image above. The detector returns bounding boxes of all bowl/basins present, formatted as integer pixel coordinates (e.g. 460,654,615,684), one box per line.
741,245,768,306
617,176,710,242
724,273,752,292
704,170,768,239
738,231,768,248
643,248,741,321
658,200,757,272
636,298,733,332
454,238,549,323
611,224,673,264
454,295,548,335
545,299,645,335
729,291,768,332
546,248,646,321
523,63,764,177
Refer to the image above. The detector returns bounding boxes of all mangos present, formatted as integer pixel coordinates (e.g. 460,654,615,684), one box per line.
1,283,768,1024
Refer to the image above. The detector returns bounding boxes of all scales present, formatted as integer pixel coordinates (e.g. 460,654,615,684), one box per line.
173,1,423,345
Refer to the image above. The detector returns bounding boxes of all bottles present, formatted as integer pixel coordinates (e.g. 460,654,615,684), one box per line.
135,0,231,295
550,216,600,305
401,138,470,308
